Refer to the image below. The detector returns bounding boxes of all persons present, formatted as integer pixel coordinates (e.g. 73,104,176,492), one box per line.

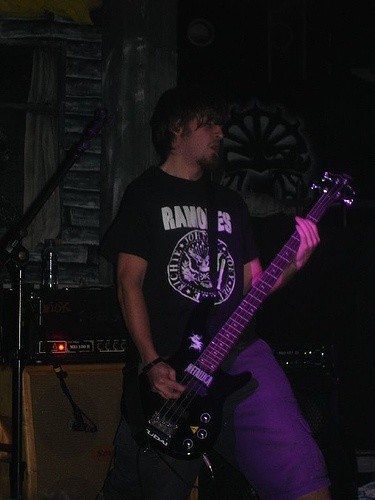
97,87,335,500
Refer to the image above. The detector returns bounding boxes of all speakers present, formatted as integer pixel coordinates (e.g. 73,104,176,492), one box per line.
199,338,344,499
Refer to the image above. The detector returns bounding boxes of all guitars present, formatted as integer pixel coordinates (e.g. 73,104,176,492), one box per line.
127,172,358,464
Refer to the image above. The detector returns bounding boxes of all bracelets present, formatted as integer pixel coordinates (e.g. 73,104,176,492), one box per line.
141,356,167,374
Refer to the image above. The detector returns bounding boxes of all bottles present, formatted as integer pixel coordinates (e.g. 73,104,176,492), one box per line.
42,238,58,291
